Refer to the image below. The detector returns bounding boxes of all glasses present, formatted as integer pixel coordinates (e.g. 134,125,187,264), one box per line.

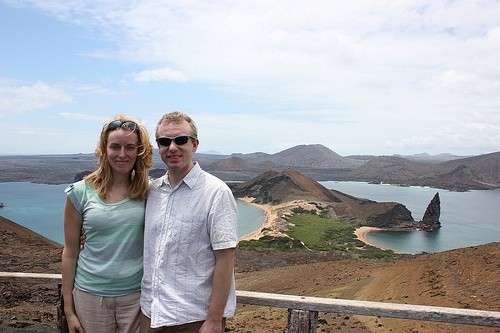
156,136,194,147
106,120,139,133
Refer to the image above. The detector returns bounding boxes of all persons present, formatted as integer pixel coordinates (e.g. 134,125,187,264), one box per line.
62,115,156,333
80,113,239,333
